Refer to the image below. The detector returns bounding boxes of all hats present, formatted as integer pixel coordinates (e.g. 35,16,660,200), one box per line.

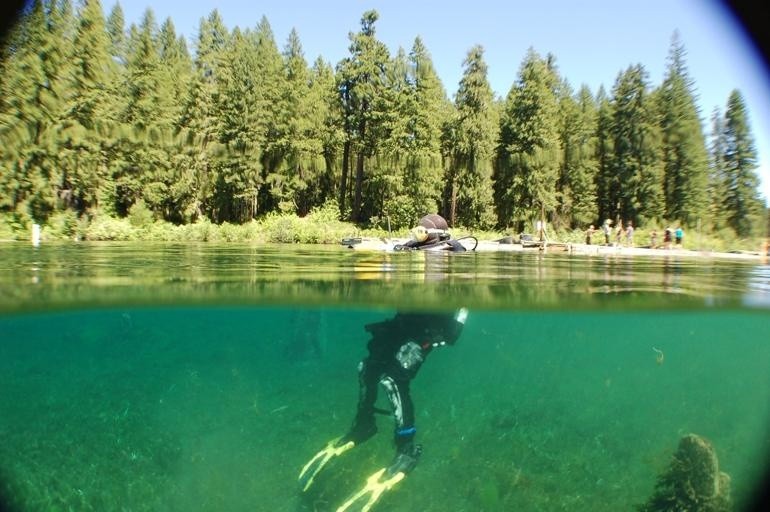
418,213,448,239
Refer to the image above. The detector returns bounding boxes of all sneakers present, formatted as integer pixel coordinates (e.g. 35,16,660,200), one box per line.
347,416,375,441
389,440,413,473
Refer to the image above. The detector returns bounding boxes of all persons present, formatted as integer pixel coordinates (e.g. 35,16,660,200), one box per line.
761,236,770,267
333,213,477,479
585,217,683,251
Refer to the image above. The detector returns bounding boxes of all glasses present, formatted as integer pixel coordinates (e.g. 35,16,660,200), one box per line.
412,226,428,243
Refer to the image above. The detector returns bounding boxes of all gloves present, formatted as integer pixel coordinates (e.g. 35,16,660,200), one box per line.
432,320,463,344
